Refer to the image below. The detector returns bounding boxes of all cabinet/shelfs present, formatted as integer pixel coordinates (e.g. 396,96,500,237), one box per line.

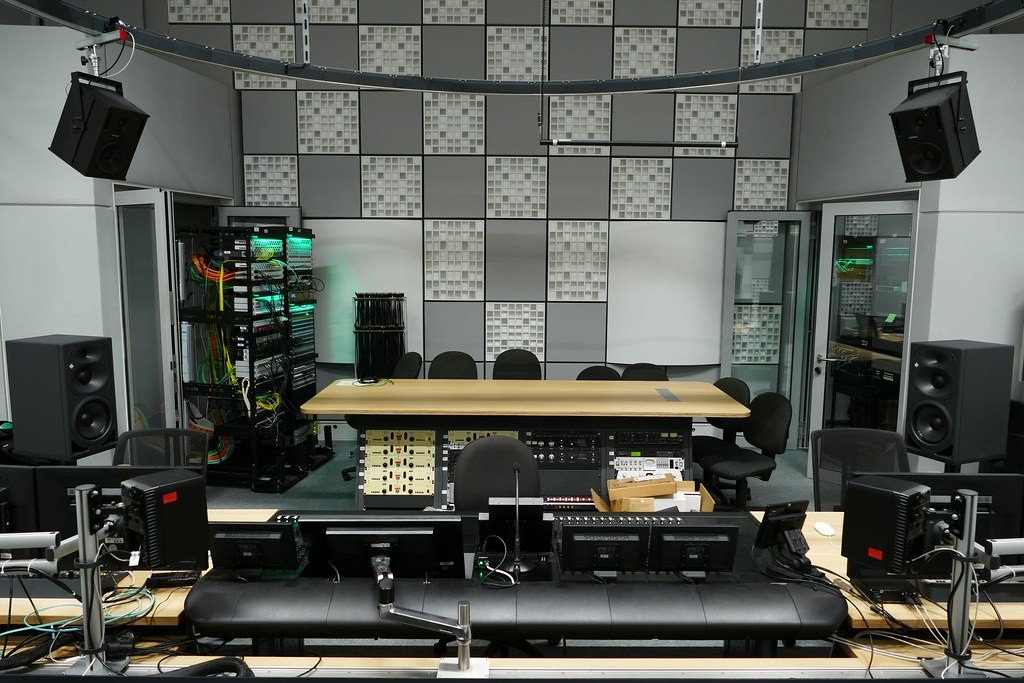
178,219,335,493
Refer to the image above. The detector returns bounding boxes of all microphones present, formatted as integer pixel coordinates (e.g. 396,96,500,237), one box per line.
497,462,537,583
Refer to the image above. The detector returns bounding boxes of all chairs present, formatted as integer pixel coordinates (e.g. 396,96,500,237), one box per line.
811,427,911,512
621,363,669,380
455,435,541,511
342,351,423,480
113,428,210,479
576,365,621,380
493,348,541,379
700,391,792,510
854,312,879,339
691,377,750,504
428,350,477,379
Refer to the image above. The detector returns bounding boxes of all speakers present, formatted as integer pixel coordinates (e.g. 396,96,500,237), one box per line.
841,473,931,581
47,80,150,182
905,339,1014,465
5,333,119,464
120,468,208,587
889,81,981,183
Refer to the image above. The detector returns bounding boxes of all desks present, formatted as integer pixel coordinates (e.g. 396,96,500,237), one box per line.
0,509,1024,682
301,379,750,511
845,327,904,352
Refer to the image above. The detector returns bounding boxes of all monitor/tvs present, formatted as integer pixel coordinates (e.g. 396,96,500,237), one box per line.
853,472,1024,580
296,514,466,581
